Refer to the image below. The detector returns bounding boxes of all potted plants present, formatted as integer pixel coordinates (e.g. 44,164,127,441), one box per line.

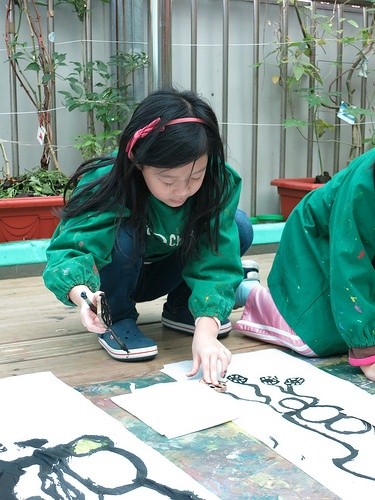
0,0,87,241
251,0,375,219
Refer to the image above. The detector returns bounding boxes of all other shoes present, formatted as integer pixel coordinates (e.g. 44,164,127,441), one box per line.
232,260,260,310
161,302,232,338
98,320,159,362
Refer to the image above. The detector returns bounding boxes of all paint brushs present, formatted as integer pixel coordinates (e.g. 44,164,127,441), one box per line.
80,290,131,355
